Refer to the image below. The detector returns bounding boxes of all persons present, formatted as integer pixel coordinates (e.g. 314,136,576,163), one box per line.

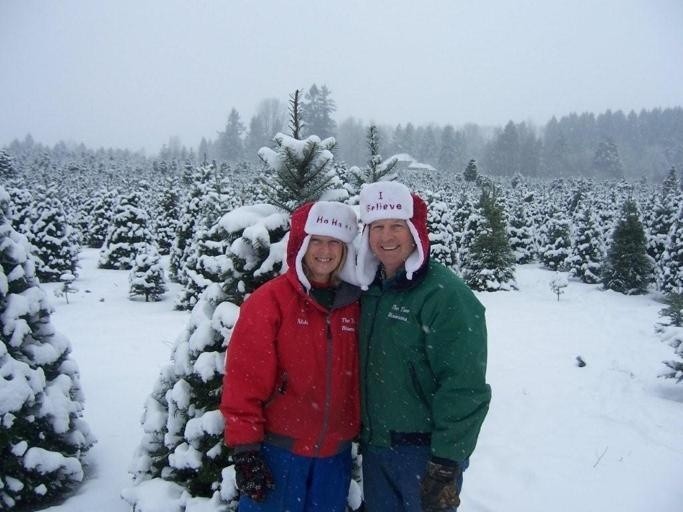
358,180,492,511
219,199,360,511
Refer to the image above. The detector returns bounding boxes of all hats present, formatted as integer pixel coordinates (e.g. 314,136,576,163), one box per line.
285,201,357,291
355,180,430,290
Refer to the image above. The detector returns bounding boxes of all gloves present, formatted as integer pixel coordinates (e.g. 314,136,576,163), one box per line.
230,451,273,502
418,461,461,511
